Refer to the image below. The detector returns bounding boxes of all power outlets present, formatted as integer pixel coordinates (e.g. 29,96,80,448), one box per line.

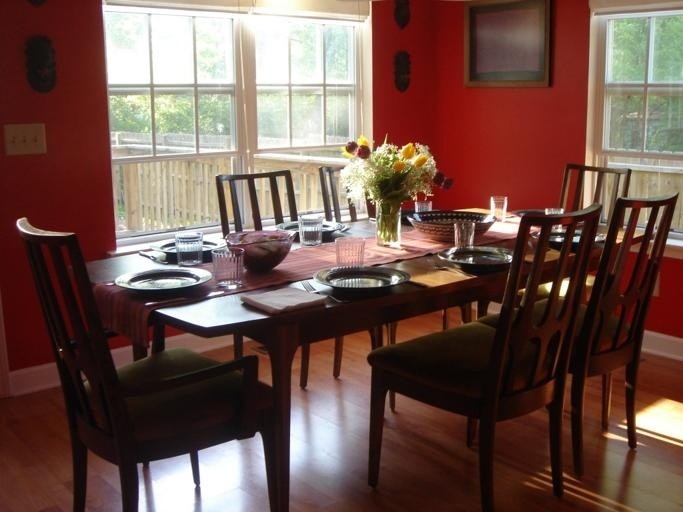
5,123,47,156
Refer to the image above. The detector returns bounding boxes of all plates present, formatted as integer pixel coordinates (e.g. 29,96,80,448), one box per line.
275,220,345,242
150,238,220,264
437,245,514,275
313,266,412,298
539,232,608,253
511,208,546,219
115,267,214,300
402,209,442,226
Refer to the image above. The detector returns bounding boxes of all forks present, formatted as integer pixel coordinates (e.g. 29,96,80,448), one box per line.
301,281,340,305
427,257,478,280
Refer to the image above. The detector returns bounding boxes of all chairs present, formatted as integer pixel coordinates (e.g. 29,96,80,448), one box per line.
477,162,632,319
213,170,343,390
466,192,678,480
16,218,281,512
319,165,452,381
366,200,602,512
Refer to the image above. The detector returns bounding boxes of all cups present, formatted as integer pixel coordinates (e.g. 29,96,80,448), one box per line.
339,267,361,288
210,247,245,289
414,201,433,211
175,231,203,267
544,207,564,232
334,237,364,267
454,221,475,247
489,196,507,223
298,214,322,245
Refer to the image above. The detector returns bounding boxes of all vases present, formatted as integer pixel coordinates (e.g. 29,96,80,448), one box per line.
372,200,402,249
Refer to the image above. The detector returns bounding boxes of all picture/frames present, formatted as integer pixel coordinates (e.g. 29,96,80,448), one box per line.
462,0,552,89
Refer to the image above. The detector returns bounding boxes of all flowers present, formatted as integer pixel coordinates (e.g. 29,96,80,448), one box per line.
335,134,450,242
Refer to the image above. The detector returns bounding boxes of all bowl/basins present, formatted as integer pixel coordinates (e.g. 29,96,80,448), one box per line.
225,230,293,273
406,210,497,243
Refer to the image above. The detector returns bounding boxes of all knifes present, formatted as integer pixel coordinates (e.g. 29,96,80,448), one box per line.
139,251,169,264
144,291,225,306
